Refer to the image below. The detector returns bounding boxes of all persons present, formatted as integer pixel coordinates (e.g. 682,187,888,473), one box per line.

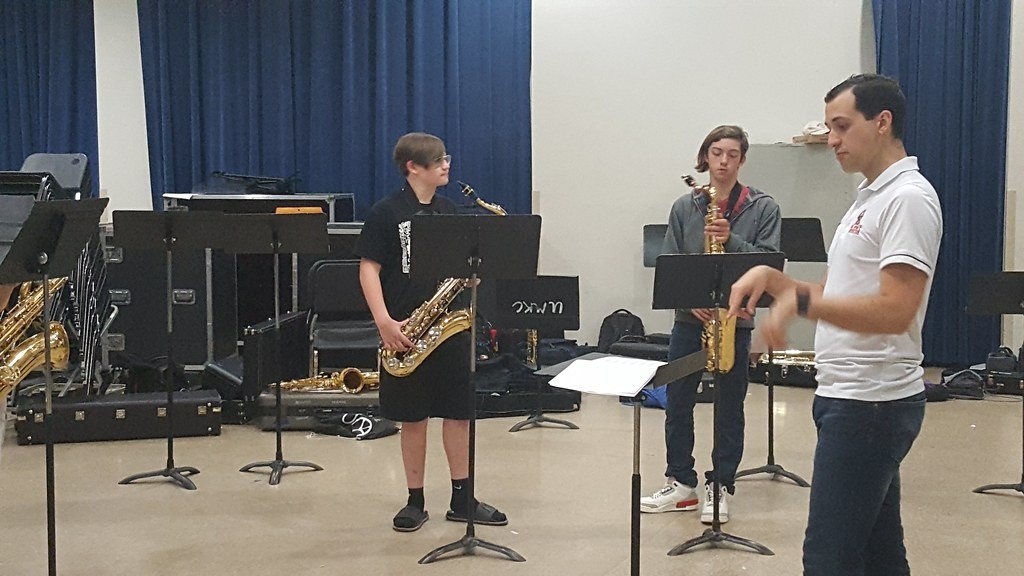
727,71,945,576
639,126,780,526
360,134,509,532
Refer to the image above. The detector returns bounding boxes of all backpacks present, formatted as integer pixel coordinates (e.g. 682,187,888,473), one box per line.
538,335,588,367
595,308,647,357
474,310,499,361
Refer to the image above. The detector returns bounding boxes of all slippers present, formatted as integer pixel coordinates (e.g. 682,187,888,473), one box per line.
394,505,431,533
445,498,508,528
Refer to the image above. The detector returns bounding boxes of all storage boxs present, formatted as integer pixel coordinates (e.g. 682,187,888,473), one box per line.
16,389,221,444
162,192,360,390
258,384,382,430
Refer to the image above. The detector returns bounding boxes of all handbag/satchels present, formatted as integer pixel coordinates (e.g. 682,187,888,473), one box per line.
942,369,987,402
925,381,948,403
986,346,1018,373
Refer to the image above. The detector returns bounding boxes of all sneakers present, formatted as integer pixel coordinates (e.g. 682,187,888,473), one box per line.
635,475,700,513
700,483,732,525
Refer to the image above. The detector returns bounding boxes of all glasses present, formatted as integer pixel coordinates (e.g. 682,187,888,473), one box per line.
416,154,452,167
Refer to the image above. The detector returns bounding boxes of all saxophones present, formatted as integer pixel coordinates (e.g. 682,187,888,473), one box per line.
270,180,509,394
681,173,741,375
0,276,70,403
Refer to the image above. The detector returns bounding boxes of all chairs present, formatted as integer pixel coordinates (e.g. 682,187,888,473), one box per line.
307,259,383,376
0,153,119,396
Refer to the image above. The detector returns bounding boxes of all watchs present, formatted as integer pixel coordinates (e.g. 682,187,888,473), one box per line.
795,285,811,317
